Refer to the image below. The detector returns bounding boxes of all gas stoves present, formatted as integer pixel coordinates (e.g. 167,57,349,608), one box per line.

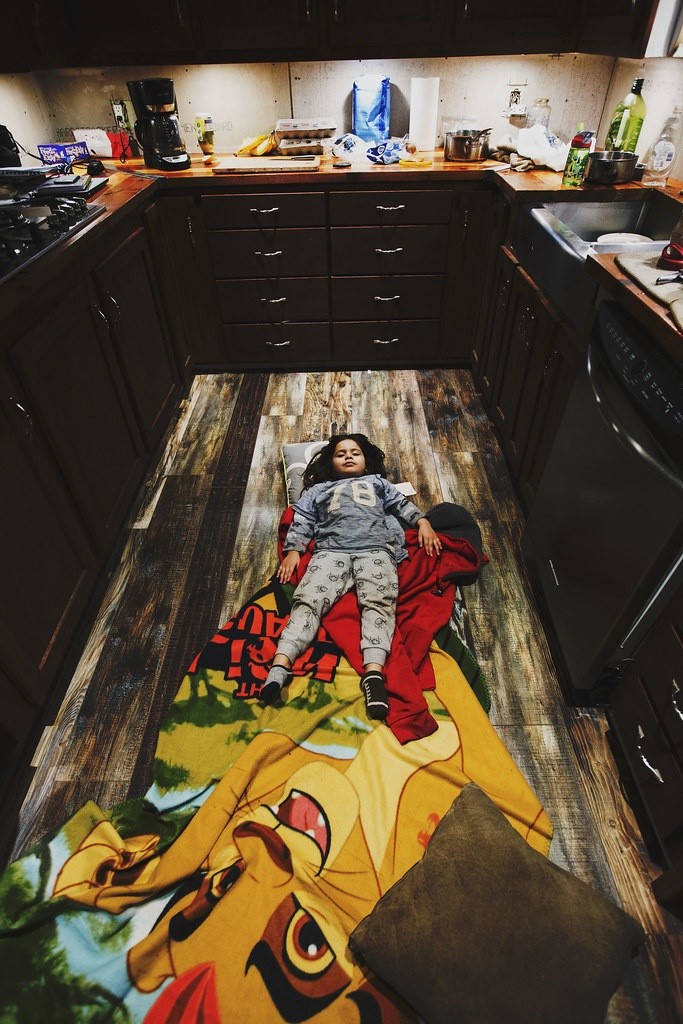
0,192,107,285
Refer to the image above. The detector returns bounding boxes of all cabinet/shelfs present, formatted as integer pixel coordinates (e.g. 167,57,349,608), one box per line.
0,154,683,880
0,0,683,73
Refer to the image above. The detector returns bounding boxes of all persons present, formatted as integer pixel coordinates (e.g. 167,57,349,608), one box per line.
260,434,443,718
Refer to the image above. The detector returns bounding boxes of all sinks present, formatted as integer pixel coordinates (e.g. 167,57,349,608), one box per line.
566,243,671,259
542,193,681,243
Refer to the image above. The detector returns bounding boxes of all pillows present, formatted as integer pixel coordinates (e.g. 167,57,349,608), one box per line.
348,780,644,1024
281,441,330,506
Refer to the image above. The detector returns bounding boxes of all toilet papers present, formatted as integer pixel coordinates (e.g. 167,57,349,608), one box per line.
408,76,440,152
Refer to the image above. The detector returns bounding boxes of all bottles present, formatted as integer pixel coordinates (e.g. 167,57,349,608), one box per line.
525,98,552,130
603,77,647,153
562,130,595,187
639,106,683,187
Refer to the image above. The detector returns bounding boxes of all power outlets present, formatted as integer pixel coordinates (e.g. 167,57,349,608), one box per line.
114,105,126,128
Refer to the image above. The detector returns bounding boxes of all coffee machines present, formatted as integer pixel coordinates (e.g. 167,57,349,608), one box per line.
125,77,192,171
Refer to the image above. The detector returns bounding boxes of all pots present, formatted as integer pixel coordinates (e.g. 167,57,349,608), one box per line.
443,127,493,163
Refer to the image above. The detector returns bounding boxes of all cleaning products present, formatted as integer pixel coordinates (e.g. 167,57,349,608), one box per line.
640,88,683,187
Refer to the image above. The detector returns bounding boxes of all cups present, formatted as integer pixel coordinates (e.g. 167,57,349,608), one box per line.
194,113,215,161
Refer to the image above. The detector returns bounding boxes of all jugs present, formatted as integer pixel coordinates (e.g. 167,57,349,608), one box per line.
146,114,185,150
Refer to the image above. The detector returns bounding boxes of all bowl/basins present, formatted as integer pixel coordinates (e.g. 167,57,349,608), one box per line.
582,150,639,185
